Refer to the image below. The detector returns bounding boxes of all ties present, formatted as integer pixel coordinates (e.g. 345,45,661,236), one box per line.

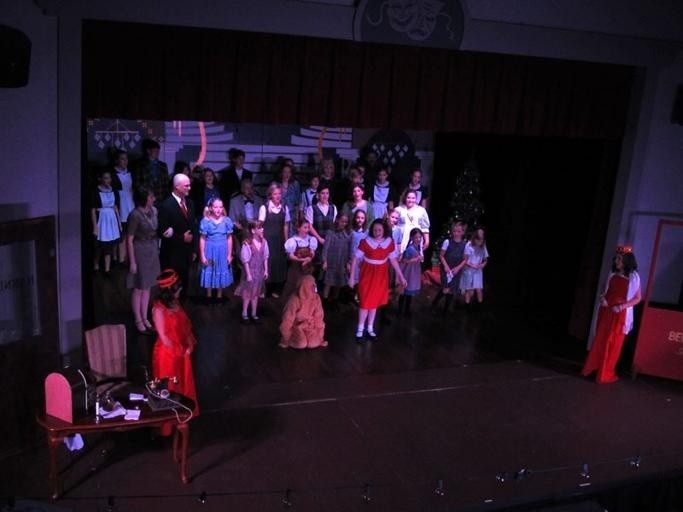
180,199,187,217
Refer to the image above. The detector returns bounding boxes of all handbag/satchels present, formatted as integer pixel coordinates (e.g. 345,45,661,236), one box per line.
146,390,184,414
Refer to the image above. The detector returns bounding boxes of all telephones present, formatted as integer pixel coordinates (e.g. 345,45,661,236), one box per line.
145,376,182,412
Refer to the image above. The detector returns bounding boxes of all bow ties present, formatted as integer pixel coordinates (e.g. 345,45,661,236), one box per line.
310,191,316,194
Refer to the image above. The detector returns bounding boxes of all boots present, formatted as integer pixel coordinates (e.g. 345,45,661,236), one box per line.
322,297,331,312
331,298,340,313
397,293,487,322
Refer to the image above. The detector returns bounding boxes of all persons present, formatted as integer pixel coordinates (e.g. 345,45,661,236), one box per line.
121,186,173,336
158,173,198,304
582,245,643,384
109,150,138,267
461,228,489,314
197,140,432,348
151,269,201,444
436,222,468,312
169,160,195,202
87,169,123,279
132,139,169,205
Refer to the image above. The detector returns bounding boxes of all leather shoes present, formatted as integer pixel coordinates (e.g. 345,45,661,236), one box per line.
367,327,379,342
355,326,366,339
142,318,154,332
249,314,264,324
352,296,359,307
240,315,251,324
133,323,152,336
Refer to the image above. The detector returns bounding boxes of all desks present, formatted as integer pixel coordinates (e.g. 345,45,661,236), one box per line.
35,401,195,502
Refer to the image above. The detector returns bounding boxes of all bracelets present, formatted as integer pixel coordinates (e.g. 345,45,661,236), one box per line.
600,297,604,302
618,304,624,311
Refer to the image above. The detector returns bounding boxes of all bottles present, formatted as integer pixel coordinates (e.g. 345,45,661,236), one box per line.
104,390,114,411
